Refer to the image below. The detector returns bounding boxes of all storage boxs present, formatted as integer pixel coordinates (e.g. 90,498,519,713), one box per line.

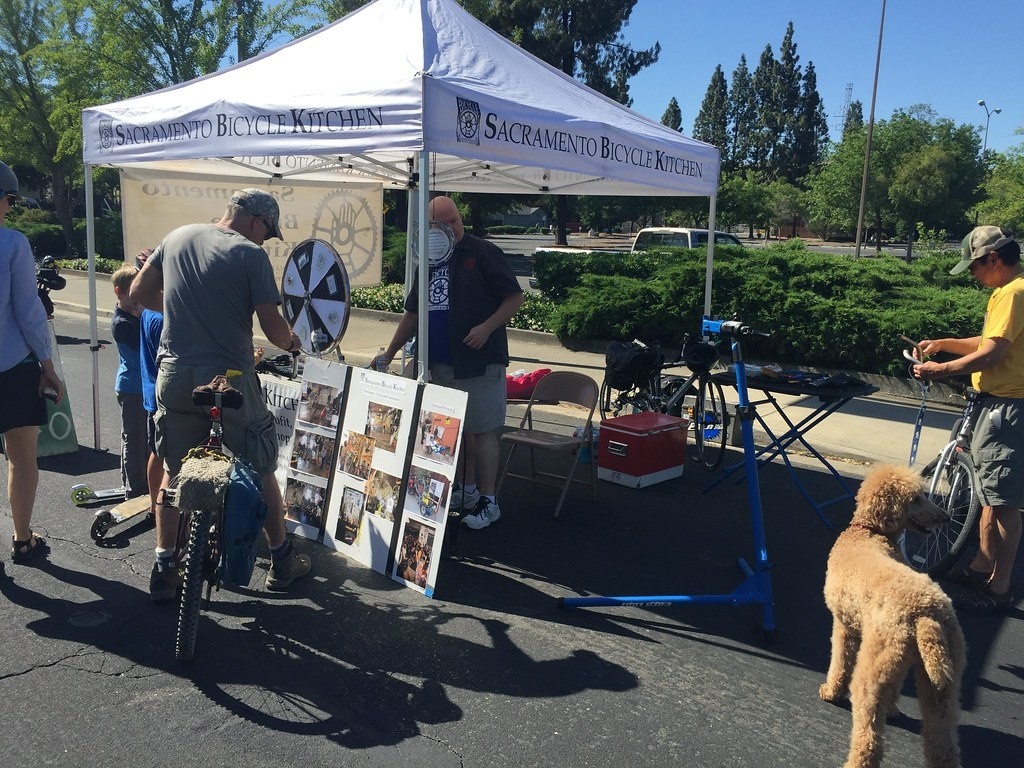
596,411,688,489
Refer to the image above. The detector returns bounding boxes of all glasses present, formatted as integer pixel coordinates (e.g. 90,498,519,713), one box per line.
263,232,274,240
5,192,15,207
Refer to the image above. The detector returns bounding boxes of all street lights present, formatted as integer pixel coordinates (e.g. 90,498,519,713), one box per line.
975,99,1003,227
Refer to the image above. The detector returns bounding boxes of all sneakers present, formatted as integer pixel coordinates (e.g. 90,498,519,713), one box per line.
449,484,480,510
460,496,501,529
264,541,311,593
149,560,183,604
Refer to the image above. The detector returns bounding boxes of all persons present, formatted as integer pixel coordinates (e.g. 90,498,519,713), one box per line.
111,250,190,546
0,161,63,572
286,386,438,589
129,188,311,599
912,224,1024,615
372,196,523,530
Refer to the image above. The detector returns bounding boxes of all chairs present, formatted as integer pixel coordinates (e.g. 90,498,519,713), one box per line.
494,368,599,519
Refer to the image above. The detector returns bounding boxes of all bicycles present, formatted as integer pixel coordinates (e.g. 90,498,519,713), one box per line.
901,348,979,572
148,342,304,661
598,330,728,471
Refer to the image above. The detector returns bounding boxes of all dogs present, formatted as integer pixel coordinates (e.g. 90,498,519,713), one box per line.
820,468,980,768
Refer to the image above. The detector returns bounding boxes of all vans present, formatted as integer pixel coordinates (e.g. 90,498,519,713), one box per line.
631,225,748,256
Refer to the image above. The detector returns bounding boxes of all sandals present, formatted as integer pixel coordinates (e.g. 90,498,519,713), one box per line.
0,560,5,573
11,528,46,561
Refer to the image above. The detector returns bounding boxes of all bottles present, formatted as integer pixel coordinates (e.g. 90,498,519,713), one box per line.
572,426,600,463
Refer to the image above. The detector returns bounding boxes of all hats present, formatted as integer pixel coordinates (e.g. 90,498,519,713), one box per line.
949,225,1014,275
232,192,284,241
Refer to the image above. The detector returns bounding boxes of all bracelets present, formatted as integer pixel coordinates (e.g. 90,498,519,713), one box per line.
285,340,294,351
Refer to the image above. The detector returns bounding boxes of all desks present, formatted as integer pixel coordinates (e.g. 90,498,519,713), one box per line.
698,372,880,532
506,399,560,430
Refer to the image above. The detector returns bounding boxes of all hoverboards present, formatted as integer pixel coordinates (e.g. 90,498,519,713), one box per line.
70,482,154,539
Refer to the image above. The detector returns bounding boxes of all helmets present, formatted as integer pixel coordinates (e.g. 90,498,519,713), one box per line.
684,343,721,373
0,161,22,201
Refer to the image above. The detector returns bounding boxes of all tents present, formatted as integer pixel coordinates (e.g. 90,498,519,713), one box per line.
81,0,722,455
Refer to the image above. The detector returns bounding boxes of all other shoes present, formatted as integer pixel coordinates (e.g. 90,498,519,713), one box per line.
946,567,992,587
953,587,1016,614
